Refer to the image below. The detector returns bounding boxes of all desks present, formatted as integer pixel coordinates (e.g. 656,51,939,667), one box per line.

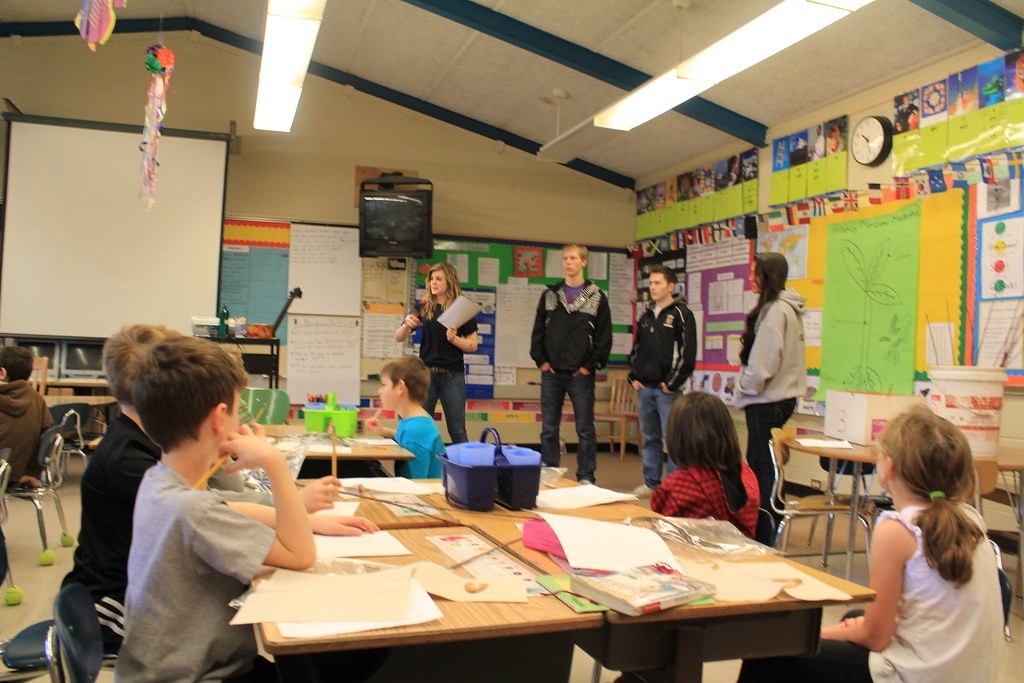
241,477,879,683
253,420,417,478
40,394,116,407
784,433,1024,601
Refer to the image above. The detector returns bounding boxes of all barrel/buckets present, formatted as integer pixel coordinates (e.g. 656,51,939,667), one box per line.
927,365,1008,457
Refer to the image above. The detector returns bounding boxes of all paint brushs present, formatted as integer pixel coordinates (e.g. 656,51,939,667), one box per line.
331,436,338,477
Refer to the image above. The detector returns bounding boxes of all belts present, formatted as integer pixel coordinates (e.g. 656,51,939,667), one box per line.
427,366,458,374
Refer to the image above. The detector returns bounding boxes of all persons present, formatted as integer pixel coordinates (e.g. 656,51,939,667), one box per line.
0,346,54,488
112,336,379,683
394,263,479,443
733,403,1005,683
734,252,807,548
366,355,446,479
71,324,343,657
529,244,612,486
614,390,761,683
627,266,696,501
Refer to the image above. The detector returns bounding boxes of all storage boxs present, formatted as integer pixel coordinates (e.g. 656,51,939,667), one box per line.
821,389,929,447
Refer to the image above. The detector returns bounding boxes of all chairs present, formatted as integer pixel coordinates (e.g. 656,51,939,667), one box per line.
595,368,643,462
13,420,75,569
50,402,92,477
242,385,291,426
973,456,999,516
28,351,48,393
767,427,873,580
0,446,24,609
46,580,103,683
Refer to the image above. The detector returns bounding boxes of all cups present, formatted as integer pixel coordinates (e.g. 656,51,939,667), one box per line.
445,440,543,466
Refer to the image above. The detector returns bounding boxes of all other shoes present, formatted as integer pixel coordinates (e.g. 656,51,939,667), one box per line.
631,484,655,499
578,479,593,485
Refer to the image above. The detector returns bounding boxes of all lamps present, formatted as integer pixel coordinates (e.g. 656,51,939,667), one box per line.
249,0,331,133
535,0,878,166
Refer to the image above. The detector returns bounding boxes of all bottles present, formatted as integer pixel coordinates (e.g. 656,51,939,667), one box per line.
219,304,229,338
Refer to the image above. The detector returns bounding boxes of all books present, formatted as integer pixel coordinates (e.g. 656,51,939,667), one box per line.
571,561,716,617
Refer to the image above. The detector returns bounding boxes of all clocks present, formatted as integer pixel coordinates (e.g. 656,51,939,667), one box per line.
850,113,895,169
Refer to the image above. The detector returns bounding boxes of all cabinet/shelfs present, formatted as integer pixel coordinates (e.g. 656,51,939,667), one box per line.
195,336,281,389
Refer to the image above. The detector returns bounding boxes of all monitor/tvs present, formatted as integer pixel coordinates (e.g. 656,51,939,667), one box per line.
15,339,59,377
359,190,433,259
61,341,104,378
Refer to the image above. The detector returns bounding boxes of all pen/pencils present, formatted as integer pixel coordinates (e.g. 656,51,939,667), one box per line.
364,409,384,431
191,452,234,490
